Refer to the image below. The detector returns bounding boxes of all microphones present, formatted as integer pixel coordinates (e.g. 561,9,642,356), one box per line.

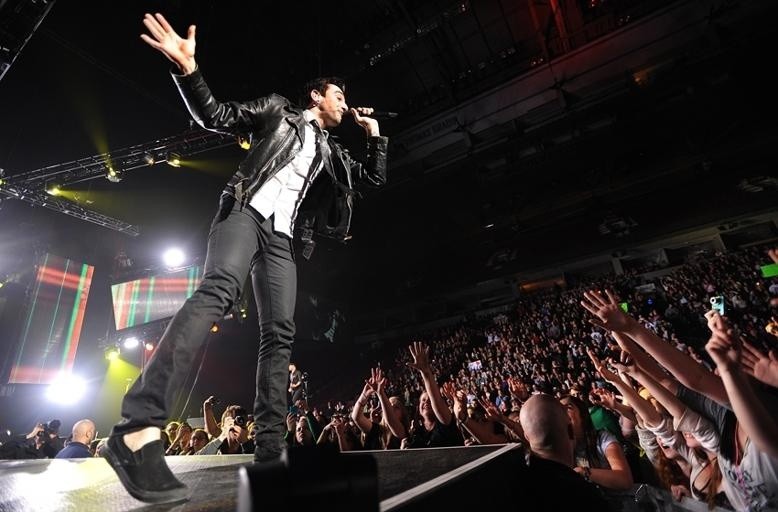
343,111,399,117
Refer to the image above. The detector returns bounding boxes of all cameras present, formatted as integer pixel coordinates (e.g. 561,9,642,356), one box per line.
229,416,248,432
37,420,60,437
710,296,724,316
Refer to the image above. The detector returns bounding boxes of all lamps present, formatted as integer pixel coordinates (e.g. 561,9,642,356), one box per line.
44,134,256,197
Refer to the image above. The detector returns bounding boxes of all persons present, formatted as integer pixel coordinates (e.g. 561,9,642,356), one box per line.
337,240,777,511
0,358,336,464
100,10,392,507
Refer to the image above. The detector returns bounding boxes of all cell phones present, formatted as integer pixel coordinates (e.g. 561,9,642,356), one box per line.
290,406,297,417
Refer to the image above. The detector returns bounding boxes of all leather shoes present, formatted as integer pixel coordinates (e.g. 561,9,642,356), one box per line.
99,433,189,504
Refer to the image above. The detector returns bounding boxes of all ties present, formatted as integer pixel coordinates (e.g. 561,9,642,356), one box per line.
310,118,363,201
